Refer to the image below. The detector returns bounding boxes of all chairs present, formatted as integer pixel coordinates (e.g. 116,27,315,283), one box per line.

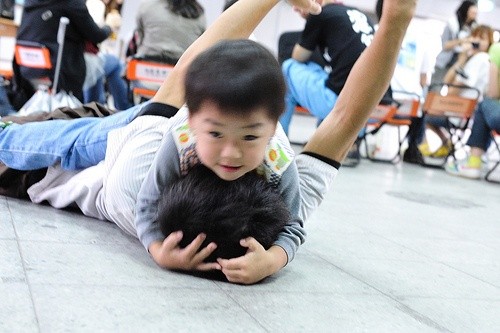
279,31,328,144
15,42,51,94
128,55,173,105
485,131,500,185
410,81,481,170
359,89,419,163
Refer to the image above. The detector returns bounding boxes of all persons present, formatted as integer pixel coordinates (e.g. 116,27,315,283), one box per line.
405,26,494,158
117,0,206,103
1,0,417,282
0,39,306,285
12,1,134,113
85,1,123,58
445,43,500,179
424,0,478,128
274,0,394,166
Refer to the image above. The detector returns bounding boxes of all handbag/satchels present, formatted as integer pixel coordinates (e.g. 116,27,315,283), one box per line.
7,76,36,111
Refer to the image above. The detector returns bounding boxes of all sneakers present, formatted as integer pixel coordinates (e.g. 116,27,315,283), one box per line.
304,137,360,167
424,142,454,162
444,157,481,178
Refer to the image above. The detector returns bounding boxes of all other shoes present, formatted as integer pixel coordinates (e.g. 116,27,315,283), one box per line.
404,147,423,164
